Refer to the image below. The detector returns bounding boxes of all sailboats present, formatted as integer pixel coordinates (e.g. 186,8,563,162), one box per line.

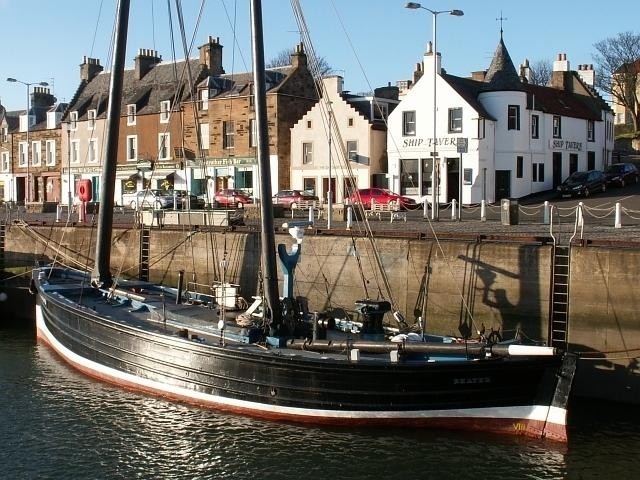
27,0,617,449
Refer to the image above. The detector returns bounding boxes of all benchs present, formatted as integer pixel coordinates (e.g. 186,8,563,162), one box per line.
290,200,325,221
363,203,408,223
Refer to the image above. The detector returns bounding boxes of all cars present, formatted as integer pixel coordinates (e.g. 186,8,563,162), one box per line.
349,188,417,212
272,189,320,211
116,187,253,209
556,170,608,199
604,162,640,188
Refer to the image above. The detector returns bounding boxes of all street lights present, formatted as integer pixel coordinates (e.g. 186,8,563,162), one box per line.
403,1,465,222
